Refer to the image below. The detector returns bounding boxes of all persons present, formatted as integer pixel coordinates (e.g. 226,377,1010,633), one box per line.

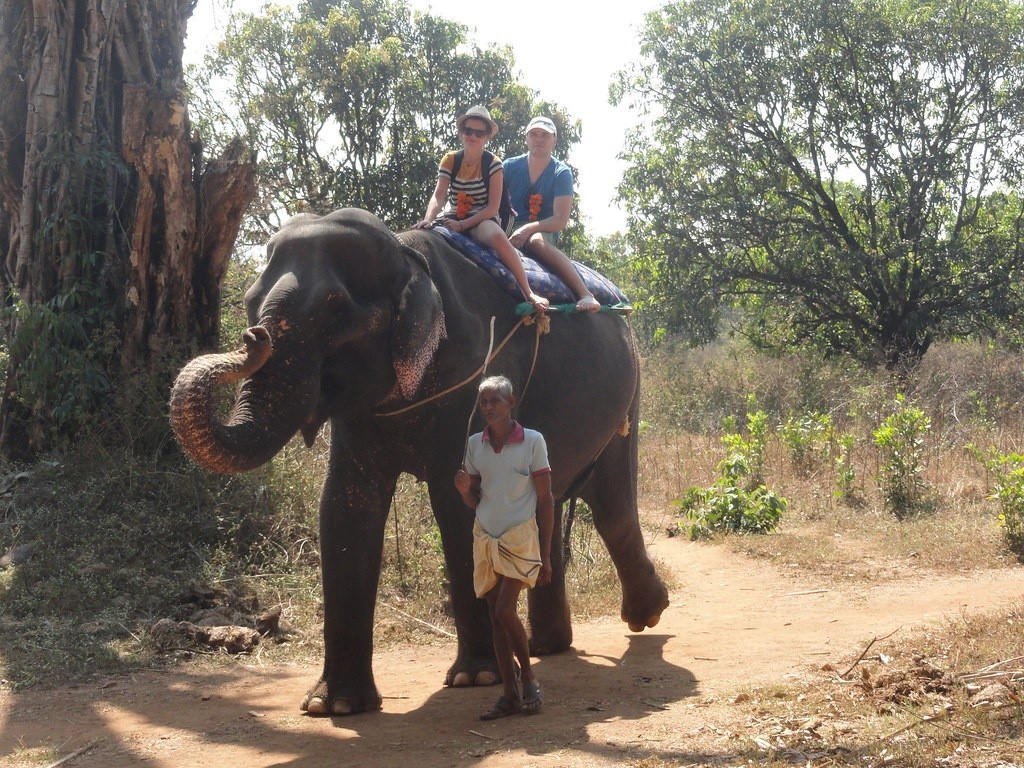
453,375,555,721
502,116,601,313
410,105,550,312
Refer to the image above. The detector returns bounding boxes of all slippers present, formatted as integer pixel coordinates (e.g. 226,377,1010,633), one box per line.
520,684,544,716
479,696,520,720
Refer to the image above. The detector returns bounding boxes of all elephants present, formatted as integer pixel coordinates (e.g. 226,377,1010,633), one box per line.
169,208,672,719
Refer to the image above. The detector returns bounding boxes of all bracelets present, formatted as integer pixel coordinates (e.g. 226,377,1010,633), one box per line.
460,220,465,233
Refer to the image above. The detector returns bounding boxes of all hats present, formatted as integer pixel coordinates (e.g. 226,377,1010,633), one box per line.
456,106,498,139
526,116,557,140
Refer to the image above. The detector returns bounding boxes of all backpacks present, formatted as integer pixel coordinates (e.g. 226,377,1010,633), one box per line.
450,149,518,239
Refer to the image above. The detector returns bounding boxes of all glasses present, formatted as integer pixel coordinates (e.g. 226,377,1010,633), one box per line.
461,127,490,139
